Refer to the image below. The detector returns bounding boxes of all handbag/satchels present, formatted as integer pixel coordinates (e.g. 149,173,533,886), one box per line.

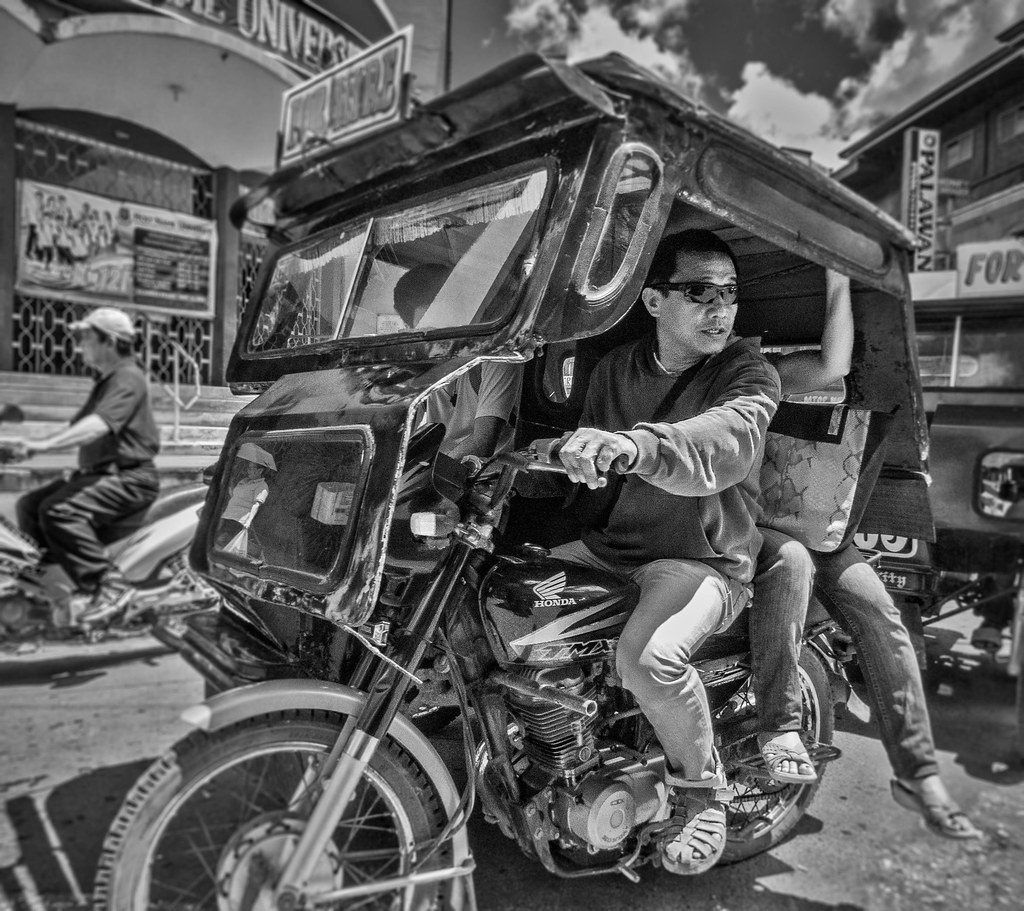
751,399,873,554
222,526,247,557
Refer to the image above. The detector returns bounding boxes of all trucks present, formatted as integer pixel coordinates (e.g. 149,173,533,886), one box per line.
824,22,1023,775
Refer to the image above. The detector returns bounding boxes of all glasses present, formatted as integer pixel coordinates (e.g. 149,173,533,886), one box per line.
654,281,740,306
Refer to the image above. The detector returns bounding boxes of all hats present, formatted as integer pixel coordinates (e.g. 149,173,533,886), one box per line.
70,307,136,343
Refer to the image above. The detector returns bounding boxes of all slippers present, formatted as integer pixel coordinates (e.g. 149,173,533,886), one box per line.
756,733,816,783
890,778,979,838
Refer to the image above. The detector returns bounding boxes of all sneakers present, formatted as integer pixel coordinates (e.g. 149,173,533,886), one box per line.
77,568,138,622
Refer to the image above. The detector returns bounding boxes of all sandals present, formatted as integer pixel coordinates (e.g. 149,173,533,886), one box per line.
659,784,737,876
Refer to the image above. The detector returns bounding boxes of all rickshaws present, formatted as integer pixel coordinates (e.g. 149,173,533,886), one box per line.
99,50,937,910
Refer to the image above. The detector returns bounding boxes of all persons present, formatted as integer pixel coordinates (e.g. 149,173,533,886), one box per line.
24,192,116,268
531,228,781,874
0,306,159,621
390,264,523,533
747,264,978,839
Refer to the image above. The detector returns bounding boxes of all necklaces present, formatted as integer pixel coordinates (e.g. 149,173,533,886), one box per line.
651,341,672,373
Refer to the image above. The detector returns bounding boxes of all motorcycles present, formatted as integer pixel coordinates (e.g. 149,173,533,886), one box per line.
0,405,222,658
